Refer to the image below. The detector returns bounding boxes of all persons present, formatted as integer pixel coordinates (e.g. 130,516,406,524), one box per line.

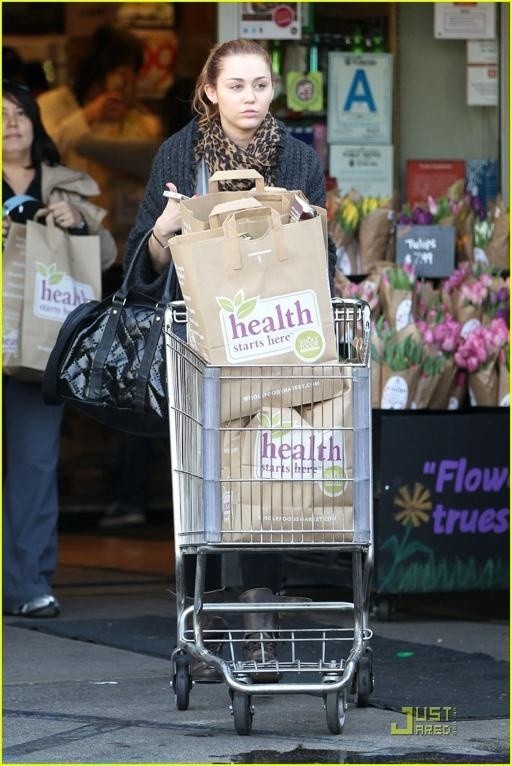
32,23,165,529
2,67,119,620
120,38,336,683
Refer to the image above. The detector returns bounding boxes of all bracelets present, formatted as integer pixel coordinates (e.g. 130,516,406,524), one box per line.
150,226,166,251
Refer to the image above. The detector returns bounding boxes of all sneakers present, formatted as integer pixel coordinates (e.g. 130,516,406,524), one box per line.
10,594,63,618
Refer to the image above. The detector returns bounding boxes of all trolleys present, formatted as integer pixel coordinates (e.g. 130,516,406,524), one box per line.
164,297,374,736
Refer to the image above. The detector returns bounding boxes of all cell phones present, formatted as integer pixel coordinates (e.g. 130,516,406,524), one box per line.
162,190,190,202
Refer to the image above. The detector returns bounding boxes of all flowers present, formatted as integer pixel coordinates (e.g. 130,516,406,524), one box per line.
326,177,510,389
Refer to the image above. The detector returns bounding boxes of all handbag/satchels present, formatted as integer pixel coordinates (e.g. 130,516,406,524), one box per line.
2,204,109,386
171,198,345,423
179,169,310,236
42,297,176,437
168,362,359,546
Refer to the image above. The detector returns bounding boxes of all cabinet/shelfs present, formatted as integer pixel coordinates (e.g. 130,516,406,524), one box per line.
371,405,510,622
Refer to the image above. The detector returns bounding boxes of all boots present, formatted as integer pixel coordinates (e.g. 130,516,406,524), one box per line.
237,587,281,685
172,586,228,685
